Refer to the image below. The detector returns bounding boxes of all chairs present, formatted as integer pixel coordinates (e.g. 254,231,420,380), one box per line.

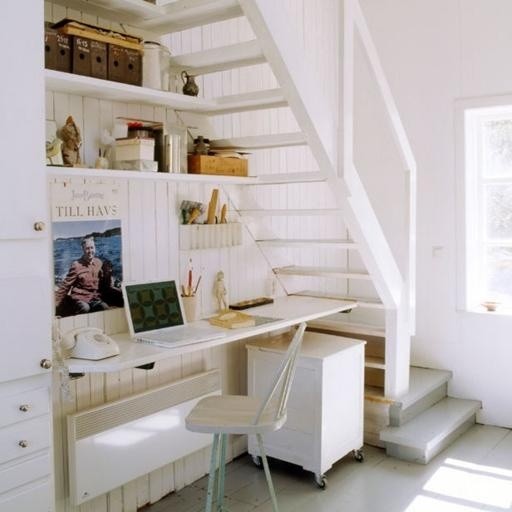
183,317,308,510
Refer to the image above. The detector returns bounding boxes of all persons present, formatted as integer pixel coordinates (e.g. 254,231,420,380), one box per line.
56,236,115,314
214,270,228,311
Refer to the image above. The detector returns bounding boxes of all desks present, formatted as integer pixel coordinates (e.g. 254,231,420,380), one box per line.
54,292,363,386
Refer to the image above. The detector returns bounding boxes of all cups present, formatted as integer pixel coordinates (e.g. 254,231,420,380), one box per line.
180,294,197,320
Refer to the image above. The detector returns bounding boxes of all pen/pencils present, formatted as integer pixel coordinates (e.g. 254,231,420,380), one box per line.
182,271,201,297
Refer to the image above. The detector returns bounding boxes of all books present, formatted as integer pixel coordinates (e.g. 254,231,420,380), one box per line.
166,135,173,173
174,135,181,172
210,309,257,329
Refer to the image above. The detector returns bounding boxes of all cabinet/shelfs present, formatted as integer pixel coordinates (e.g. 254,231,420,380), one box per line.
243,328,369,490
42,1,256,249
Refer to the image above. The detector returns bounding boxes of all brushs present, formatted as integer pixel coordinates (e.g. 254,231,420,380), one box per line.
187,201,207,226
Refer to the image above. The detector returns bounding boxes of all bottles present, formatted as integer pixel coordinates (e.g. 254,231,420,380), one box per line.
181,70,199,96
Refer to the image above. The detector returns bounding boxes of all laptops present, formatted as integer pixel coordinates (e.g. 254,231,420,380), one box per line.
121,279,226,349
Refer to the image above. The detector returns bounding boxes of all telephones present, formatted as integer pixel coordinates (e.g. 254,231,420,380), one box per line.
63,327,120,360
